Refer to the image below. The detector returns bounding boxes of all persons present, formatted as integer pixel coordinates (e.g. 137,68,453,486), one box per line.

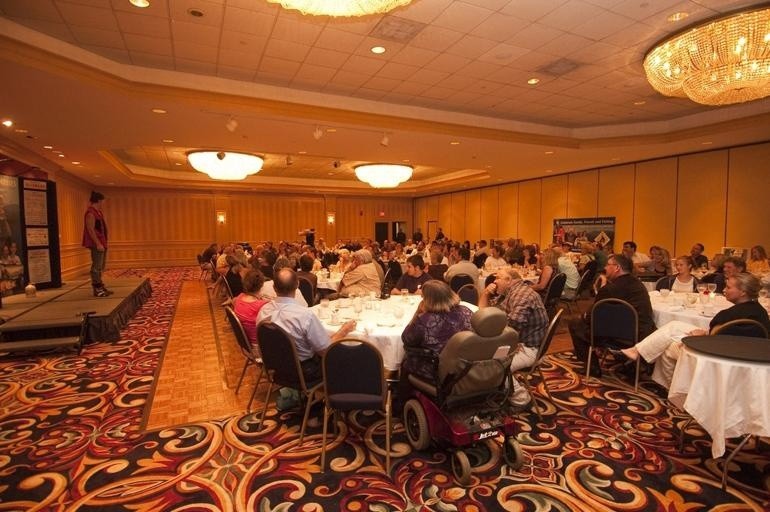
390,254,432,295
608,272,770,391
248,228,387,275
658,256,699,293
423,249,448,281
748,245,770,277
633,246,672,278
81,191,115,298
576,244,595,271
381,228,470,266
689,243,708,269
530,249,559,301
515,245,537,269
532,243,539,256
400,280,476,385
553,247,581,300
568,254,656,378
443,247,478,284
484,246,506,271
607,249,614,258
221,270,271,363
714,256,746,294
255,267,357,385
494,239,524,261
562,243,573,257
594,242,608,273
472,240,491,263
477,269,549,413
258,257,309,307
701,254,725,283
327,249,381,301
296,255,321,306
0,241,24,294
622,241,650,264
200,243,252,298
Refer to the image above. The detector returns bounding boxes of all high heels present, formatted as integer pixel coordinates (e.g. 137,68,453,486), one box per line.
608,348,635,367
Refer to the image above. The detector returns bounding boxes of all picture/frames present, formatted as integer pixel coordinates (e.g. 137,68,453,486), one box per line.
552,217,615,254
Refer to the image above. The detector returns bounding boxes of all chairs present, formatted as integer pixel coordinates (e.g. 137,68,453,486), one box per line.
669,273,700,292
458,284,479,307
209,260,218,280
225,304,286,411
544,273,567,323
320,338,390,476
514,309,565,420
197,254,213,281
586,297,655,393
510,257,518,264
255,320,338,446
485,273,505,305
657,276,668,290
298,276,314,307
596,275,608,295
221,275,234,299
452,274,474,293
711,319,768,338
428,264,448,280
558,269,592,316
381,267,393,296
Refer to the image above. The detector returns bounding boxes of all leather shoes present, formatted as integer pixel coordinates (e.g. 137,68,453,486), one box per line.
572,367,602,378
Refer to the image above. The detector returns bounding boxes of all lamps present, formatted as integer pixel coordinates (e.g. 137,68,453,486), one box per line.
379,132,389,147
313,125,324,140
185,149,264,181
353,163,414,190
642,2,770,105
329,217,334,226
266,0,414,18
130,0,151,8
188,7,205,18
334,160,341,168
218,151,225,159
287,156,293,165
225,115,238,133
668,11,690,23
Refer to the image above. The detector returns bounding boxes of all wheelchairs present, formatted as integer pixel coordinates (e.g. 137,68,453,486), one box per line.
397,307,526,484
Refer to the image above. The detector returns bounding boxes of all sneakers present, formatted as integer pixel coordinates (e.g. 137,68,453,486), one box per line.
93,287,105,296
507,401,533,415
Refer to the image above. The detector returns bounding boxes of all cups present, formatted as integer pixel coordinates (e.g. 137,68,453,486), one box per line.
696,284,708,294
708,283,718,293
703,304,712,315
401,288,408,299
698,311,716,317
660,288,669,299
354,301,362,317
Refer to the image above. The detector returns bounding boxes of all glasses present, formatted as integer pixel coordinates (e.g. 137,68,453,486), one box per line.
607,263,617,266
